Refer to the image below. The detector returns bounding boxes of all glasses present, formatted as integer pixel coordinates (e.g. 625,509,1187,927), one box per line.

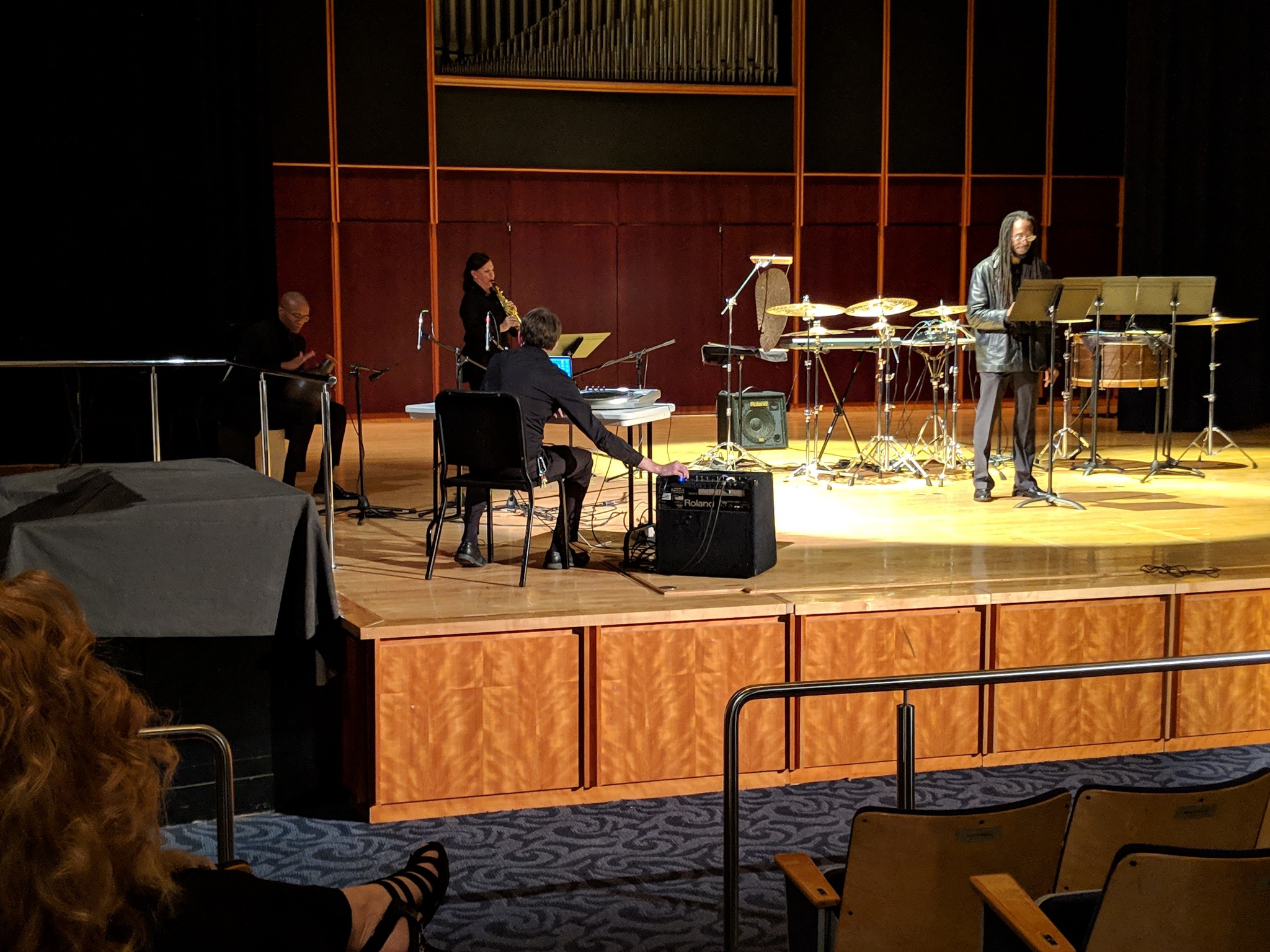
1012,235,1037,242
285,310,310,322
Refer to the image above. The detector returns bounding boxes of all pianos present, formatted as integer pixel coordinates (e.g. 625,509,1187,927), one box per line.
779,336,900,472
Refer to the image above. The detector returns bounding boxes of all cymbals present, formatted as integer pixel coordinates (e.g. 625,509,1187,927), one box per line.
910,305,968,316
845,298,918,317
765,302,846,318
1169,313,1260,328
900,333,977,346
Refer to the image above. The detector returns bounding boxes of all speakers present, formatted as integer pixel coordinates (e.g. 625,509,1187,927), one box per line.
716,386,788,449
655,470,778,578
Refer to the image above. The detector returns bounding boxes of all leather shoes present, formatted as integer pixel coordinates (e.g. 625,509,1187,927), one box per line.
542,549,591,571
453,541,487,568
312,481,361,500
973,488,992,502
1011,483,1057,499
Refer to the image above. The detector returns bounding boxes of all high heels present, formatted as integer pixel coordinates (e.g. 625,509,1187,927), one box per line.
360,840,450,952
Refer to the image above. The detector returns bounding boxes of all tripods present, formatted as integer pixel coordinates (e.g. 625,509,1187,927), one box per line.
1007,279,1088,512
1130,277,1216,483
1062,275,1138,479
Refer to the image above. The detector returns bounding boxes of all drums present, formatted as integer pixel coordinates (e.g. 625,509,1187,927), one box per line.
1071,333,1172,390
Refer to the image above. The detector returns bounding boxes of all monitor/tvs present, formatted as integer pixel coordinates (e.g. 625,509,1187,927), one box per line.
550,355,573,381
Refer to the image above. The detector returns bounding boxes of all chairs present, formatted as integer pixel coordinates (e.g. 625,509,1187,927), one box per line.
768,788,1073,951
967,843,1270,952
423,389,570,587
1054,764,1270,890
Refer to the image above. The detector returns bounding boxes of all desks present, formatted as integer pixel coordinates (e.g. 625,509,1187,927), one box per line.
405,399,676,567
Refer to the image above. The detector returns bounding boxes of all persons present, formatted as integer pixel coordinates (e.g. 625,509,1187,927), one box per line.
236,292,365,503
964,210,1066,502
458,253,525,392
454,307,690,570
0,572,457,952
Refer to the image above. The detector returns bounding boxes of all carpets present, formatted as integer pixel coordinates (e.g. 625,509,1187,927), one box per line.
150,741,1270,952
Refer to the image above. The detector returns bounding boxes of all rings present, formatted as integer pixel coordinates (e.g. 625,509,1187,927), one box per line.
513,321,515,324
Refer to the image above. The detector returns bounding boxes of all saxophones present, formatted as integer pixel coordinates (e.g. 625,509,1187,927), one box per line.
489,280,523,331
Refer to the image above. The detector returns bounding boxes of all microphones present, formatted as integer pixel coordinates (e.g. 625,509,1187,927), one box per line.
416,312,424,352
485,312,491,352
368,367,393,381
